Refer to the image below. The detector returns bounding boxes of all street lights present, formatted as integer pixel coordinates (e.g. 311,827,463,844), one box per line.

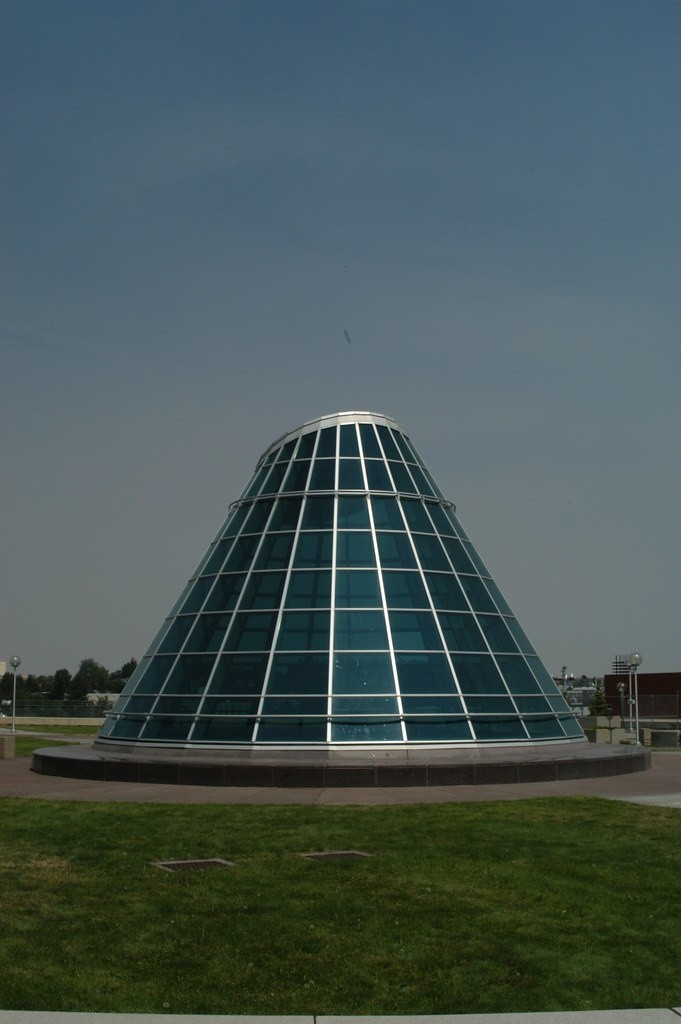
617,682,627,729
9,655,22,734
625,656,635,733
627,653,642,746
